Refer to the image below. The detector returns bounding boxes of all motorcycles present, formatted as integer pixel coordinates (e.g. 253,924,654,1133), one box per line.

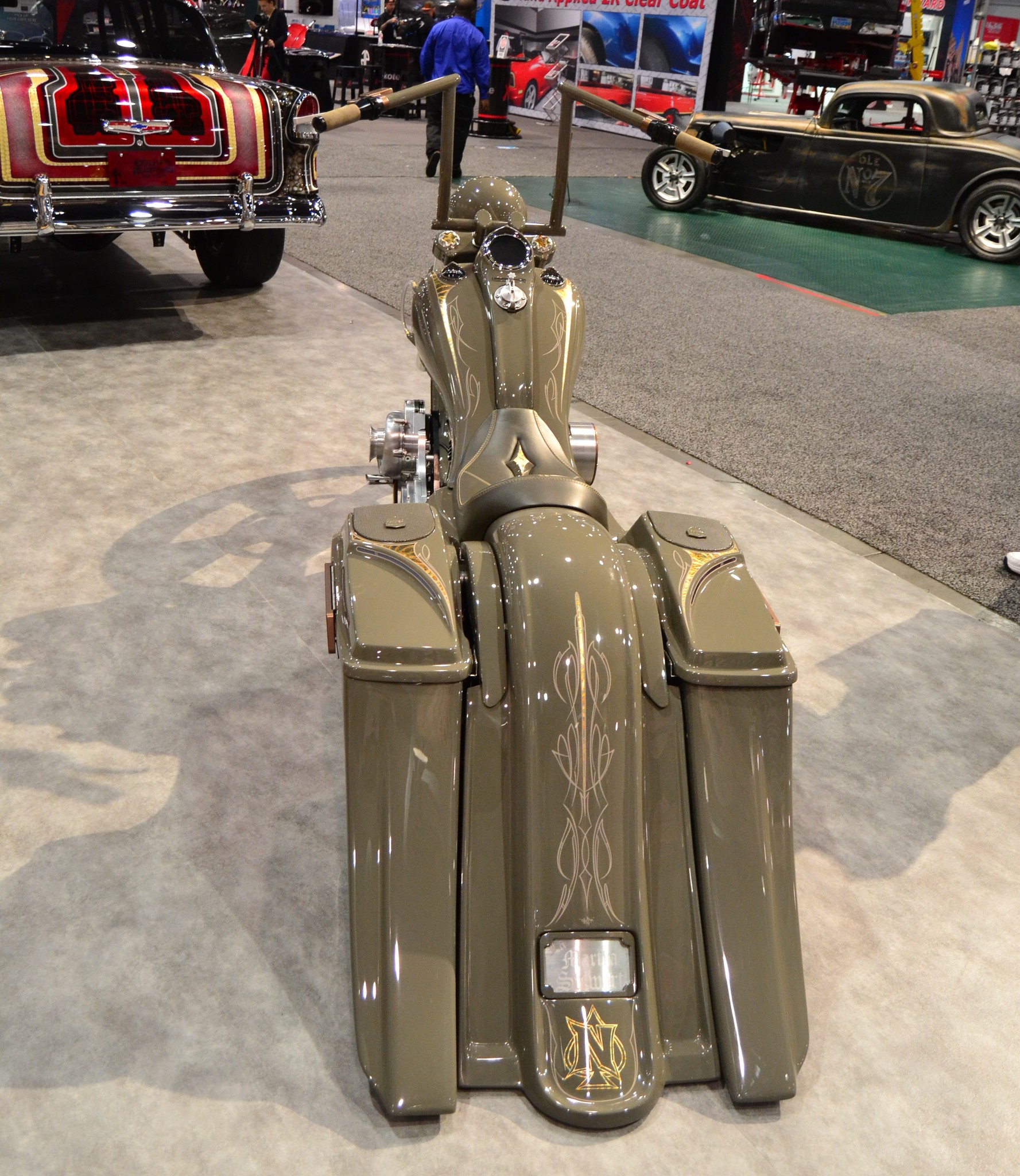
298,73,815,1116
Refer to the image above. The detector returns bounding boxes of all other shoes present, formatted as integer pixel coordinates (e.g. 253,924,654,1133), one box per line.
452,163,461,178
426,152,441,177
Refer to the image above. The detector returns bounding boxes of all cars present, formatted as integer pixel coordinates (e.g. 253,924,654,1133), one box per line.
0,0,325,294
641,80,1019,259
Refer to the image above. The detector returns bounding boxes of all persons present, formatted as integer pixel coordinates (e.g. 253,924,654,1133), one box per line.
421,0,493,180
377,0,404,45
247,0,288,83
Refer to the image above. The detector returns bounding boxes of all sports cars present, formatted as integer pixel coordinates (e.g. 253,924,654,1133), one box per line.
511,31,697,125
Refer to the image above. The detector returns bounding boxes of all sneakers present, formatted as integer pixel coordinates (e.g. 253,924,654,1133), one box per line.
1003,550,1020,577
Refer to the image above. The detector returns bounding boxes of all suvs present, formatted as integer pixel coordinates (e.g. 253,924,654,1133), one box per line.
751,0,906,65
